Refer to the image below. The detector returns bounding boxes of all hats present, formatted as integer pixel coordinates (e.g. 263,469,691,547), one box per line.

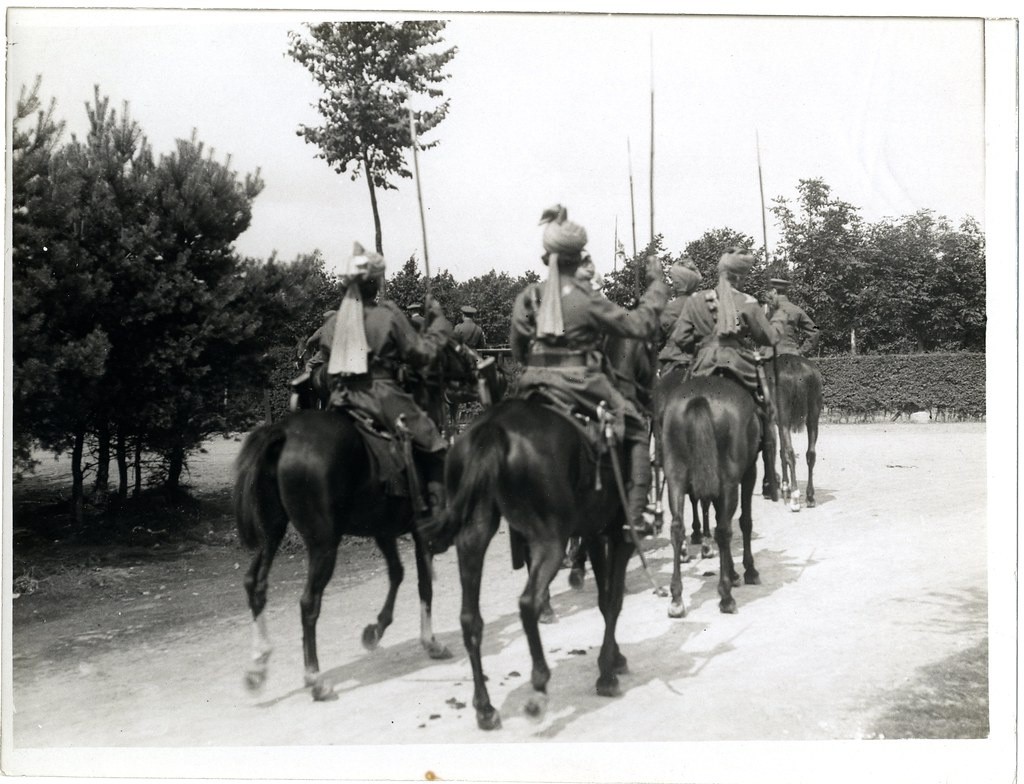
327,242,386,374
769,278,791,291
670,258,701,292
407,304,421,311
575,249,595,281
460,306,477,316
716,248,754,336
537,203,587,339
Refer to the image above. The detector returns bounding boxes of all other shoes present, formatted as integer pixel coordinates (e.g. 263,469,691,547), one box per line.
763,473,780,495
623,521,650,542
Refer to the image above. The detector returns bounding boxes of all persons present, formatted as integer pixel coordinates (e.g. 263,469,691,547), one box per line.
319,244,453,521
506,205,666,542
658,261,703,335
757,273,822,360
291,301,488,393
667,249,781,499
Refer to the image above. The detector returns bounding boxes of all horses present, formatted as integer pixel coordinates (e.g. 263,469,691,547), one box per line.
231,354,824,730
